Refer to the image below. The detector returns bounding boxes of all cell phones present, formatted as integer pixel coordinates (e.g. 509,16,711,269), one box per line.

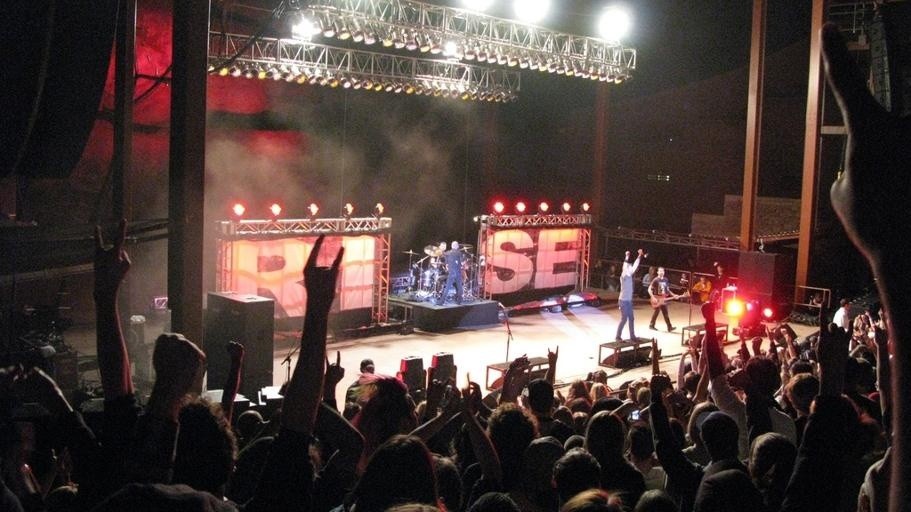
631,410,639,421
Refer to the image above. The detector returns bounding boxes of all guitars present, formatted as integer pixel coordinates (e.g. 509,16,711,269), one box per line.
651,290,690,308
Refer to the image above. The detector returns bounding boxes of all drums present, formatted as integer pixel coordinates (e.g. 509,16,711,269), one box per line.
435,276,458,302
431,262,445,275
422,271,434,287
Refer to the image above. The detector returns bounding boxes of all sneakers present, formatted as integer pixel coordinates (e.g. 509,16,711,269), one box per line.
631,337,641,343
616,337,624,344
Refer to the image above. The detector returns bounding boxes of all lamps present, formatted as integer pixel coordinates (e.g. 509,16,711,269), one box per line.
360,78,371,89
207,64,217,76
726,299,742,318
251,65,264,80
325,72,337,87
227,64,239,77
475,41,485,62
453,39,463,58
518,50,528,68
403,29,417,51
536,53,547,71
492,88,501,102
268,202,281,221
561,202,570,214
241,64,252,79
302,68,315,84
391,80,401,94
371,78,381,91
466,86,476,100
546,53,555,73
500,90,508,103
528,51,537,70
336,73,351,89
262,63,272,78
493,201,504,215
342,202,353,218
402,81,412,94
590,62,598,79
486,43,496,63
495,45,507,65
284,11,314,40
306,202,318,219
431,81,440,97
484,87,493,102
572,58,582,77
614,67,623,84
440,82,448,98
290,66,304,84
314,70,325,87
269,66,280,80
442,37,455,57
579,202,590,214
319,11,335,38
278,64,290,80
218,63,227,76
508,90,517,103
449,83,457,99
363,22,375,44
554,55,564,75
464,39,474,61
415,32,430,52
507,48,517,66
372,202,384,216
411,80,422,95
624,69,632,81
582,61,589,78
607,65,615,82
562,57,572,75
429,33,442,54
421,80,431,95
380,78,391,92
347,18,363,42
393,28,404,48
332,15,349,39
515,201,525,216
538,202,548,215
762,306,773,322
476,87,485,101
232,204,245,221
309,10,323,35
379,25,392,47
350,75,361,89
599,63,607,81
458,86,468,100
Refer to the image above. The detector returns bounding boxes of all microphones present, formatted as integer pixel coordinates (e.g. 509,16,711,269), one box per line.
678,277,690,287
498,301,512,318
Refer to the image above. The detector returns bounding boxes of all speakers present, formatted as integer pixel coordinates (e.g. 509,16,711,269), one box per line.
738,250,787,295
207,292,275,392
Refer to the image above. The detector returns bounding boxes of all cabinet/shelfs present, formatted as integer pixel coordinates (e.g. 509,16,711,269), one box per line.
680,323,728,346
598,337,652,369
485,357,555,391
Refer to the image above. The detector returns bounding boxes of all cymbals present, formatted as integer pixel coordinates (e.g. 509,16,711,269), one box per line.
460,246,473,251
402,250,418,255
424,245,442,257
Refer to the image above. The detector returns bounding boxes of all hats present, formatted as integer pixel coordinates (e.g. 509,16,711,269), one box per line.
361,359,374,369
732,355,781,389
840,298,848,305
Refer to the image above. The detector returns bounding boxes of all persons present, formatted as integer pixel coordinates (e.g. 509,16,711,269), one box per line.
606,265,620,292
346,360,375,402
616,249,643,343
424,241,446,288
649,374,751,512
220,341,244,423
651,338,661,374
710,262,729,302
873,325,892,442
411,382,501,512
735,328,819,374
436,241,463,306
585,412,646,510
92,215,343,512
544,346,558,386
347,437,441,512
552,448,601,511
0,363,102,512
648,267,679,333
820,22,911,512
464,403,538,482
422,368,451,421
679,272,689,286
628,422,666,492
502,355,530,403
683,402,719,466
692,276,712,304
786,374,820,442
124,333,205,486
849,303,887,357
833,298,851,332
523,380,573,441
351,376,417,442
700,300,796,475
567,370,651,419
643,266,657,297
678,349,697,408
809,292,823,315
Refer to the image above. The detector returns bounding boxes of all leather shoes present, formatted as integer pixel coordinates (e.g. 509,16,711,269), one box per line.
650,326,657,331
668,326,676,331
